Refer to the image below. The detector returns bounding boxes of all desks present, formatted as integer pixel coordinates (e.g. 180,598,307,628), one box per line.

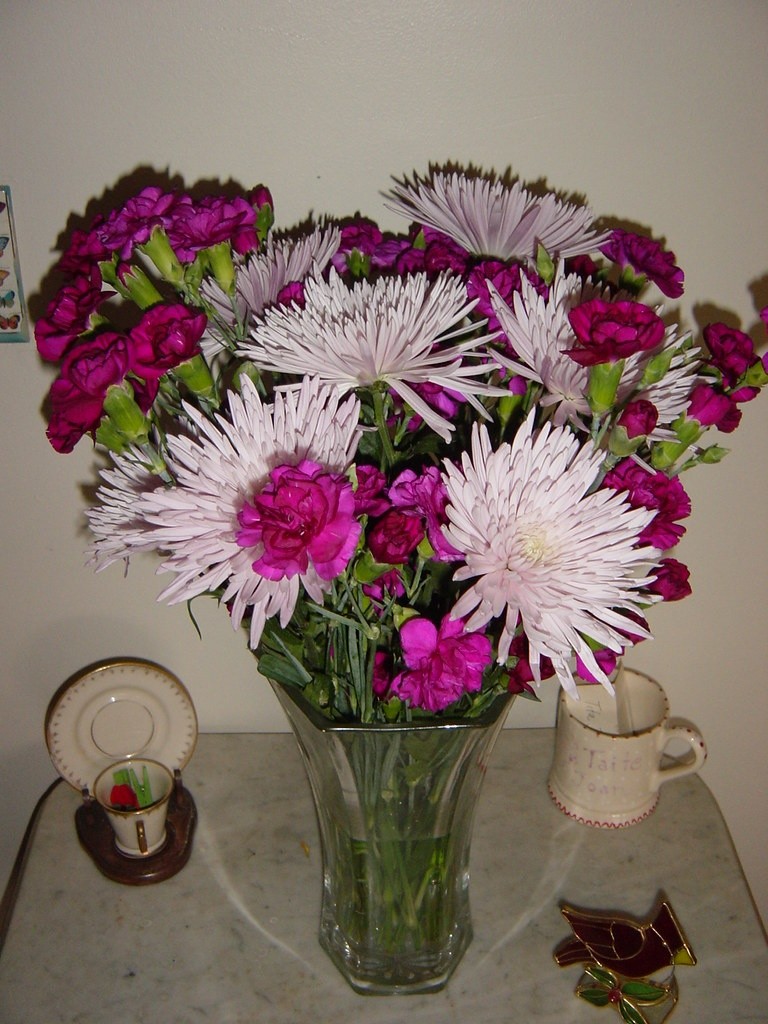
0,726,768,1024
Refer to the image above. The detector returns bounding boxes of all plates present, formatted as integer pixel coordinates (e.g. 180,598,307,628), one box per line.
46,662,199,797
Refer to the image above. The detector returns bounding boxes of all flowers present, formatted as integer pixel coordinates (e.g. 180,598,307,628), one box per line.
31,170,768,723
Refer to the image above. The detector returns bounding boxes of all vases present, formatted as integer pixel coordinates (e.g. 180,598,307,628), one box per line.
247,641,518,997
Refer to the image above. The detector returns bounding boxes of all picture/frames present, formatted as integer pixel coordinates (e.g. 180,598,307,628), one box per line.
0,186,30,343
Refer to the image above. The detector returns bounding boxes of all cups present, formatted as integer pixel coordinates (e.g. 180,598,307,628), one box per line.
94,761,174,859
547,667,707,830
